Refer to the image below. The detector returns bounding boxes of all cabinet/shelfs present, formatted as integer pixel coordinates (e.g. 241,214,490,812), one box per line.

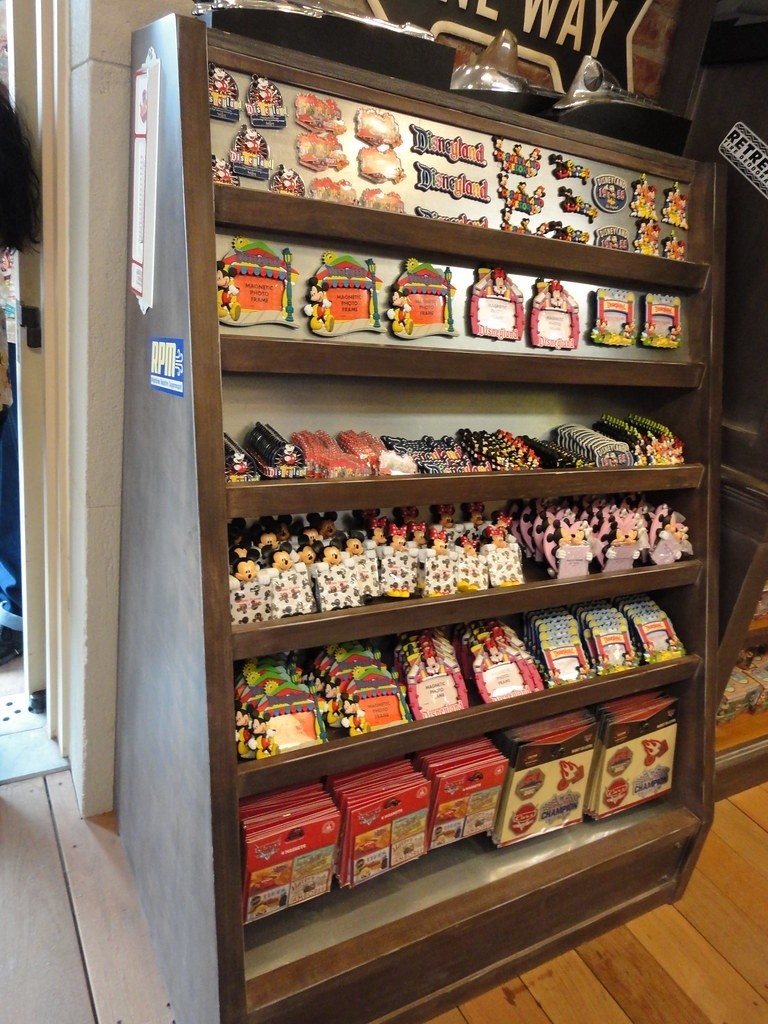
106,10,735,1023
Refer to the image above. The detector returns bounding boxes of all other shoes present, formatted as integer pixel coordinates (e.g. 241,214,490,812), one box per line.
0,625,23,665
